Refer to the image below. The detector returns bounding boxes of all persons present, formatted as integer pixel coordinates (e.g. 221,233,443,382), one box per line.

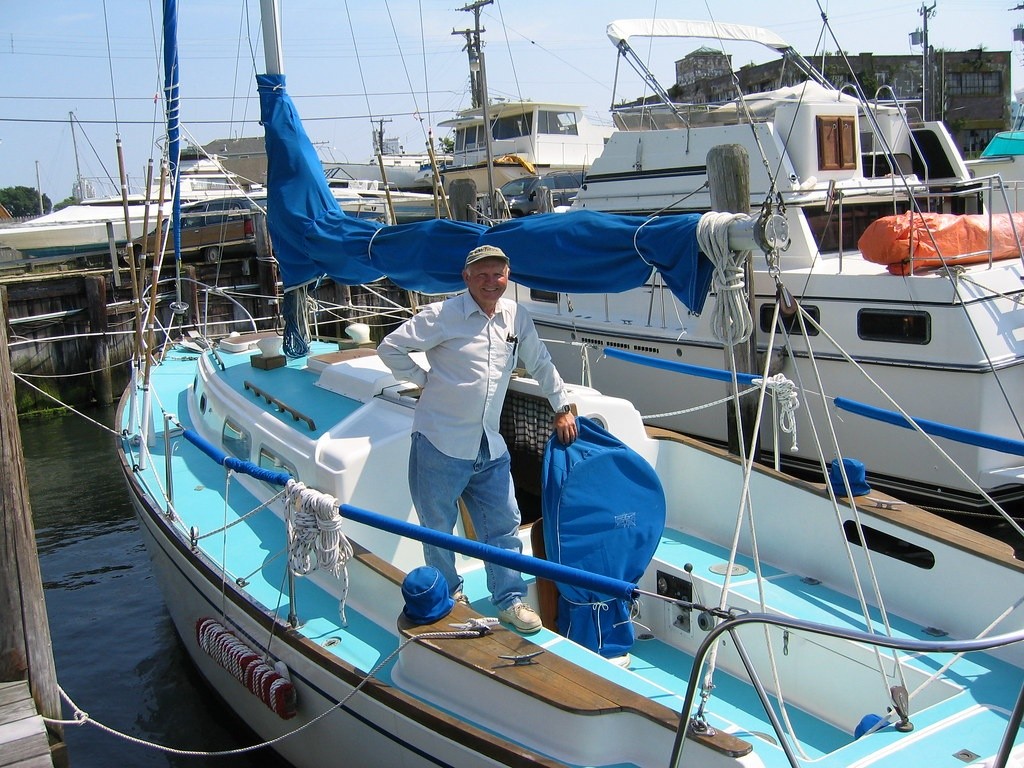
377,245,578,635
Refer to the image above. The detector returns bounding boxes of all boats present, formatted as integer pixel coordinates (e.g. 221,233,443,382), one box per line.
0,0,1023,509
110,133,1024,768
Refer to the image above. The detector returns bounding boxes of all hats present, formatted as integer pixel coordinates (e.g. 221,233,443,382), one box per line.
465,245,510,268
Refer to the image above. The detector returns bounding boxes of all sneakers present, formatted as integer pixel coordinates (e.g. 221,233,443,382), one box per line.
453,591,470,608
498,601,542,634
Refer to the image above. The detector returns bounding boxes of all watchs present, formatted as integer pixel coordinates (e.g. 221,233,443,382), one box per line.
555,404,571,414
